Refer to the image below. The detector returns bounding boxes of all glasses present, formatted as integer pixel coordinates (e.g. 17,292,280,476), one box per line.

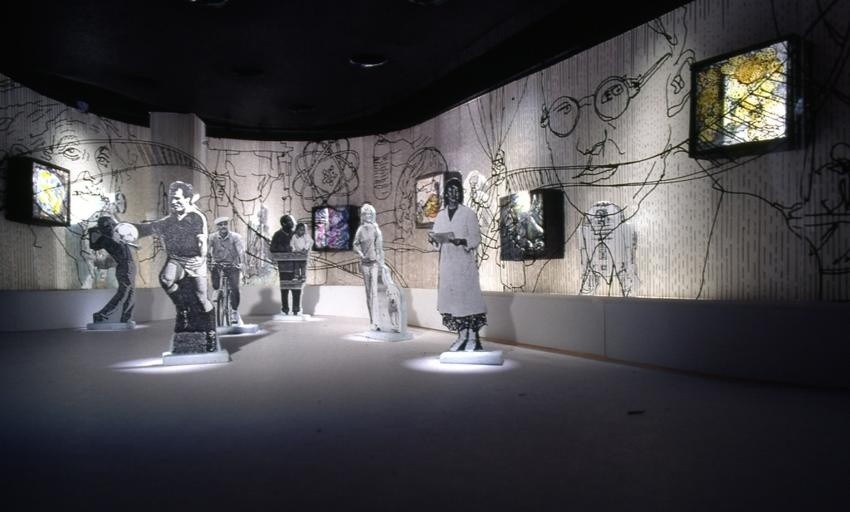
539,51,672,138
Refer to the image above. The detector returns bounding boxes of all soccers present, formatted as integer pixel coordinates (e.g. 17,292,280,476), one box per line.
112,223,138,244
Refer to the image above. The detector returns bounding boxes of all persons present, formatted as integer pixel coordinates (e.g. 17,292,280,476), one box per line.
289,223,314,282
1,75,147,290
206,217,245,324
531,5,823,303
427,177,488,352
88,217,136,324
208,174,237,233
270,215,303,315
352,203,385,331
492,149,510,232
128,180,217,352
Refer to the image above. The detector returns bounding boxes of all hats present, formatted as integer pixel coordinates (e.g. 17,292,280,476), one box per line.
212,216,229,225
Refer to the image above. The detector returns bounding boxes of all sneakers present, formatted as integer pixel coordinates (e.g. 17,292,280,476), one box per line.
94,312,108,323
210,289,219,302
205,325,218,353
231,311,238,325
174,304,189,332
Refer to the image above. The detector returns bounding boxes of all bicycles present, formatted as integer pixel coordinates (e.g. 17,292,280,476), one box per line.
207,260,238,327
